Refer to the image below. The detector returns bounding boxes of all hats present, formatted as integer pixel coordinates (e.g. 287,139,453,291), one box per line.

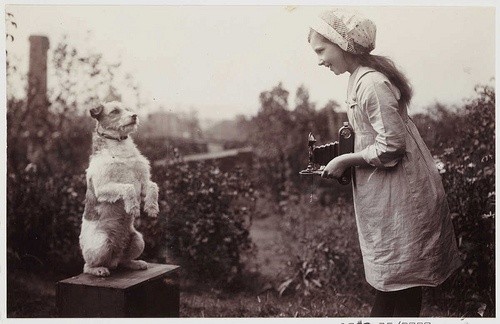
309,9,377,56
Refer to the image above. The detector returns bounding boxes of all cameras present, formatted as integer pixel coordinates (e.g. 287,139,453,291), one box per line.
301,123,355,187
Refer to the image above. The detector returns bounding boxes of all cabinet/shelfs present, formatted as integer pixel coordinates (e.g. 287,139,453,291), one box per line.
55,263,180,317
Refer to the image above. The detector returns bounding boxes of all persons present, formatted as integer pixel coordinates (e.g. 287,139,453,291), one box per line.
307,8,463,317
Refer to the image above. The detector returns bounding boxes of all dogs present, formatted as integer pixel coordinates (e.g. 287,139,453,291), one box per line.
78,100,160,276
